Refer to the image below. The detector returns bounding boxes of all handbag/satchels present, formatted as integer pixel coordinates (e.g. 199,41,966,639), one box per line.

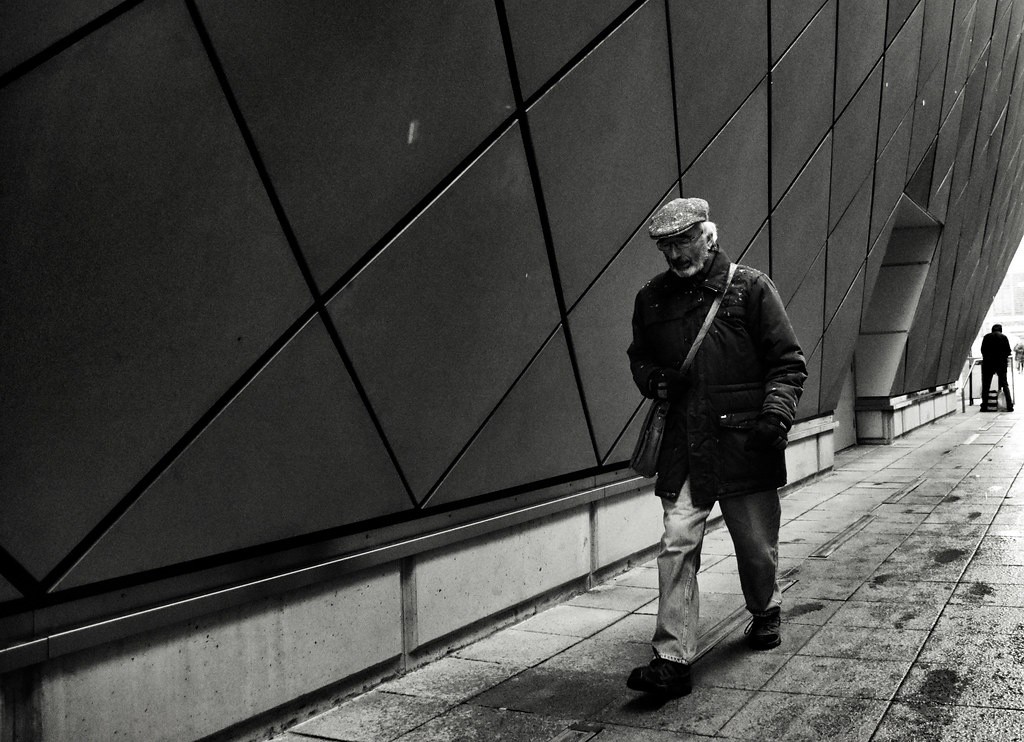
630,398,670,479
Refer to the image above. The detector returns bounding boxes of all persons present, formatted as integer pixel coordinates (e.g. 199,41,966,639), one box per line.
1014,343,1024,374
625,197,811,696
979,324,1013,413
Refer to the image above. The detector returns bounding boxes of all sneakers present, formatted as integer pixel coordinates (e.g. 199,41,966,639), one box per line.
743,605,781,649
627,646,693,692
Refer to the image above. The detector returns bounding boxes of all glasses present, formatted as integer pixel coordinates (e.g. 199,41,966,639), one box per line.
656,231,706,251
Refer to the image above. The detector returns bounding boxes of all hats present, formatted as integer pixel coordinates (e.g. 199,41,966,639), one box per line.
648,197,709,240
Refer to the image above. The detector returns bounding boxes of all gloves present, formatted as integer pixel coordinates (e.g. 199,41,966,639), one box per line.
742,414,789,464
645,369,684,403
